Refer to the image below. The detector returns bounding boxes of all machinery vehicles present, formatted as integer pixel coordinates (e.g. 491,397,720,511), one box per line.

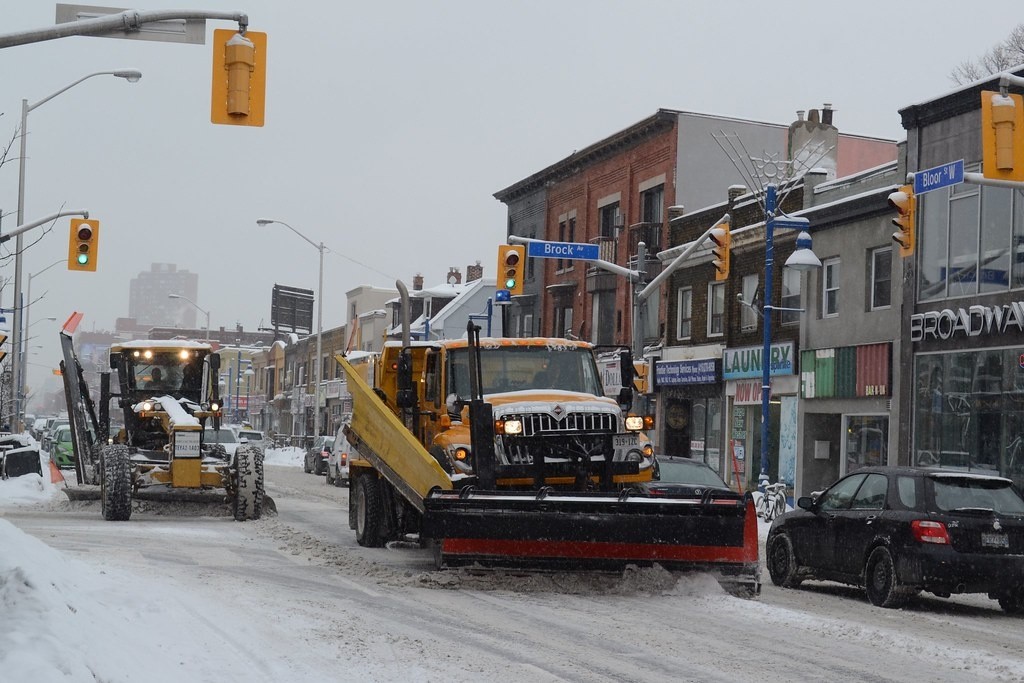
57,311,266,522
337,281,762,597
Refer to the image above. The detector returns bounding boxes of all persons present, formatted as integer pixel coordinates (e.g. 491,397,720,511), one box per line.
143,367,171,391
319,429,323,436
268,428,278,448
305,427,314,451
535,358,569,389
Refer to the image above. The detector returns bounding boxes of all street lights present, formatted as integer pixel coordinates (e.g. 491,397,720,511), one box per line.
168,294,210,344
219,351,255,423
757,184,824,493
10,72,143,434
24,316,56,411
487,290,513,337
257,219,325,444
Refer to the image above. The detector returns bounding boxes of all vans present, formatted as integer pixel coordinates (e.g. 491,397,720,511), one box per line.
238,430,265,461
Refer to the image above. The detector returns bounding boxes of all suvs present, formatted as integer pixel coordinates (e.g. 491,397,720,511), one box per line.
324,419,362,487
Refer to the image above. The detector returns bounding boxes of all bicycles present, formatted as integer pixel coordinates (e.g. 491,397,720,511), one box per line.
755,482,788,523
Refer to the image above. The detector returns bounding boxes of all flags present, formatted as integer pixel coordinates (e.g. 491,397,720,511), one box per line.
63,312,84,333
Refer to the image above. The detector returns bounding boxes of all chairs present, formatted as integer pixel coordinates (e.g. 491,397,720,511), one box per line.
869,479,885,505
453,362,468,393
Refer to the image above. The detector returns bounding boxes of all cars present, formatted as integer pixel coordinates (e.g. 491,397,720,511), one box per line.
23,413,120,469
304,436,336,475
623,455,737,497
765,467,1024,609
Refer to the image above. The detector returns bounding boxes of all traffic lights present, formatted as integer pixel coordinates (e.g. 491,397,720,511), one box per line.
68,217,99,271
708,222,730,281
497,245,525,296
0,335,8,362
886,184,916,259
